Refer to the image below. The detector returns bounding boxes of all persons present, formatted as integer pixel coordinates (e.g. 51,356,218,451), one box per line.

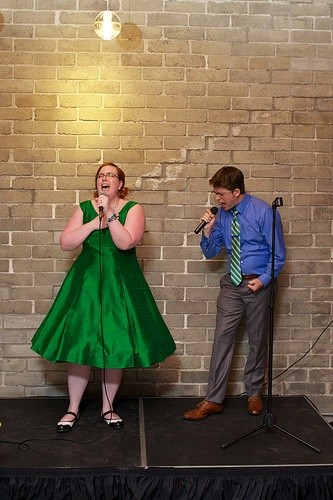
182,166,286,420
31,162,176,432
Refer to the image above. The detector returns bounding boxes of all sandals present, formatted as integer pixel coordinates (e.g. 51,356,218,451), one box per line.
57,409,80,432
99,408,123,429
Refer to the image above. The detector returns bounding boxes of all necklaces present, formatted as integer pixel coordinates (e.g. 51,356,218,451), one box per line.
112,198,119,211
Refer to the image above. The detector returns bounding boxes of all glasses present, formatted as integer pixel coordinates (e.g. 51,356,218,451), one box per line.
95,172,120,180
213,190,232,196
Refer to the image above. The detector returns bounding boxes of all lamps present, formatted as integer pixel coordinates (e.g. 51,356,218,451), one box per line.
93,0,121,41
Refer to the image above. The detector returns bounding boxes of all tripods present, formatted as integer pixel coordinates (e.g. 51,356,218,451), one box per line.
221,197,322,454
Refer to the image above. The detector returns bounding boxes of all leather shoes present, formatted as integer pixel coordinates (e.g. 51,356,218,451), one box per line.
247,393,264,415
183,399,223,420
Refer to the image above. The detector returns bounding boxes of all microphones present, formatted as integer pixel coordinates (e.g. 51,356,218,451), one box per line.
98,192,107,220
194,207,219,235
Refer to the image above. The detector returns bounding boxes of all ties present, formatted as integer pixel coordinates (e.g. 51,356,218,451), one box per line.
229,208,244,286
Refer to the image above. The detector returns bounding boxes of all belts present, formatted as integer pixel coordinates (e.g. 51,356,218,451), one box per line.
243,275,258,278
